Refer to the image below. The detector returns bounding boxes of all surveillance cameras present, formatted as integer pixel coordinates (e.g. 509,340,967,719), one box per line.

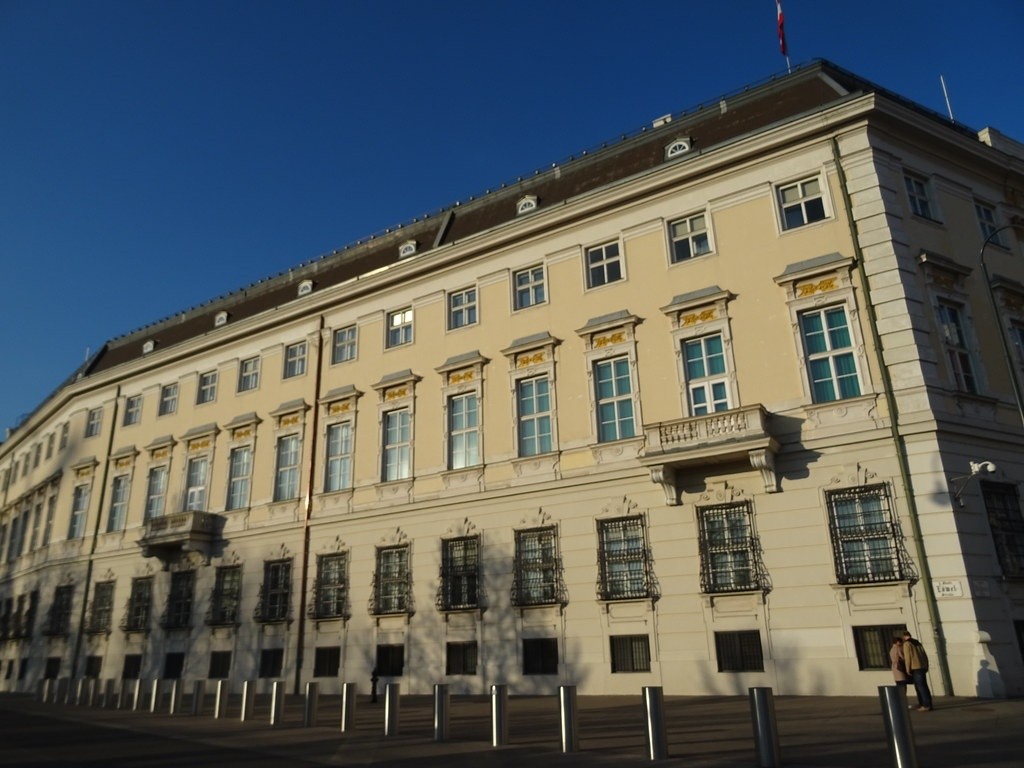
987,464,996,473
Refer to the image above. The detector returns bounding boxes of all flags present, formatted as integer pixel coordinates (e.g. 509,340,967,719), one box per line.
775,1,788,57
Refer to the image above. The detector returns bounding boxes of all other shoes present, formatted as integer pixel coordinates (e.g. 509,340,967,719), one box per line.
917,706,931,711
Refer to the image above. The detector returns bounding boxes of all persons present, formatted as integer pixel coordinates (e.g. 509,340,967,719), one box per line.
891,636,913,710
901,631,932,712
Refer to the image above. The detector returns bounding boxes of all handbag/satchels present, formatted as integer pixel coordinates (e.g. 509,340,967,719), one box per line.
898,656,906,672
